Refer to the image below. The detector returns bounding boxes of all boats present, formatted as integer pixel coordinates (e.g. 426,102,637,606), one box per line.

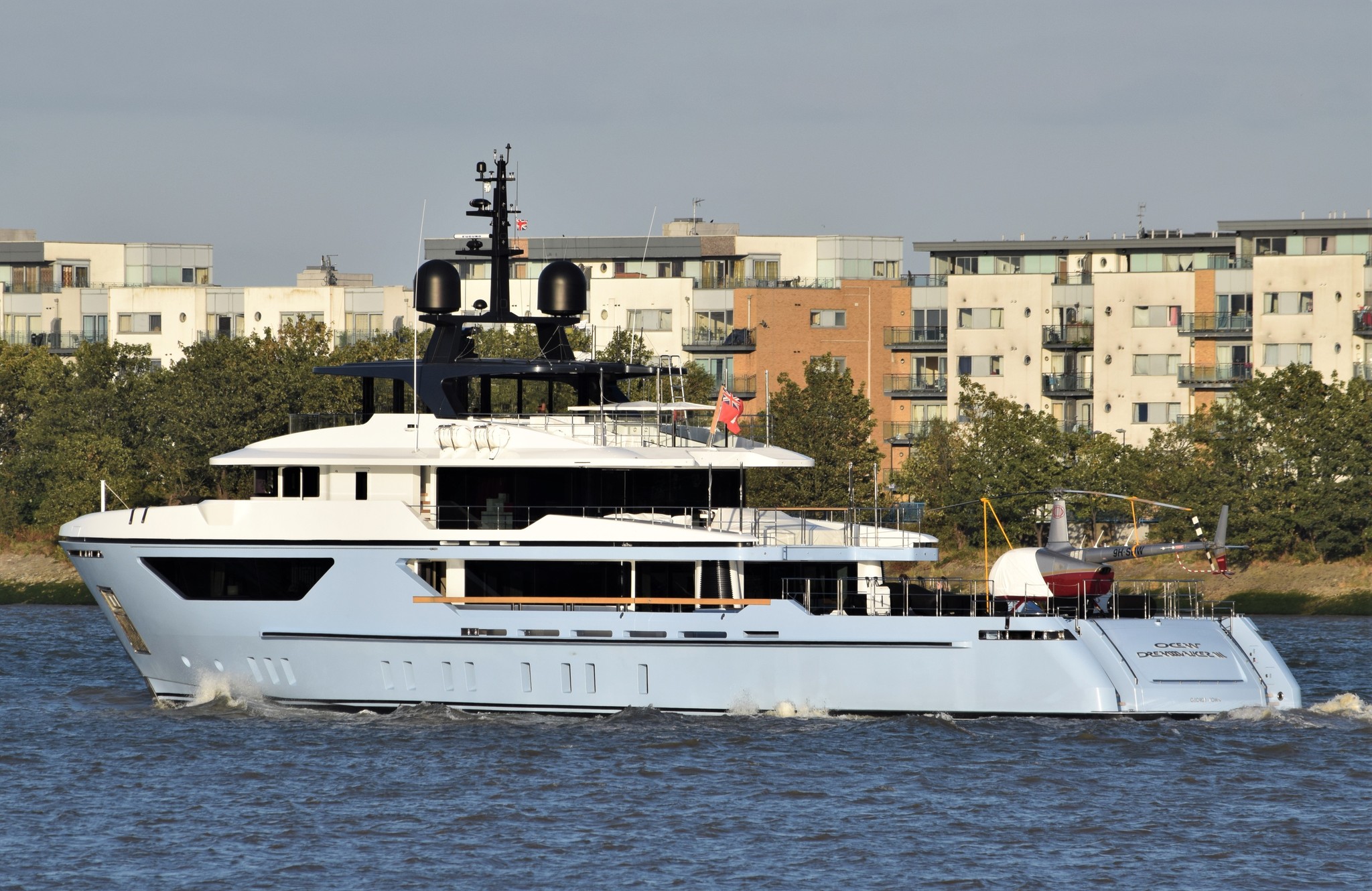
60,143,1303,725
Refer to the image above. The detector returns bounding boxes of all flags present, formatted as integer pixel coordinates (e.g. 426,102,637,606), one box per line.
719,388,744,434
516,218,528,230
